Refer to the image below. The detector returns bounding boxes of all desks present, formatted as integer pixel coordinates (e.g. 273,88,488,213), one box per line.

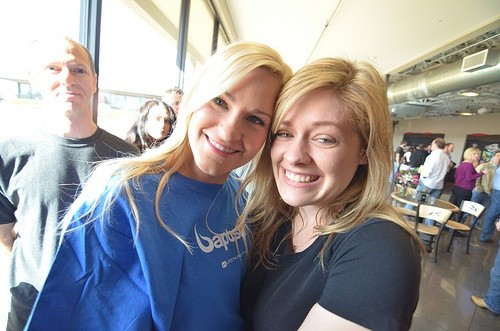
390,193,457,250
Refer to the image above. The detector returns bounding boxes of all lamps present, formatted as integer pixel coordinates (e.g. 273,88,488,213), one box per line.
459,106,475,115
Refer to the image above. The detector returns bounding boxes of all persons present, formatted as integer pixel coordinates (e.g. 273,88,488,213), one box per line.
123,86,184,154
217,57,429,331
22,40,293,331
471,215,500,316
388,137,500,254
0,36,140,331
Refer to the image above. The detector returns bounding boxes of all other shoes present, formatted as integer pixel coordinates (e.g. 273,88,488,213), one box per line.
472,294,499,317
426,244,432,253
476,226,482,231
443,227,464,237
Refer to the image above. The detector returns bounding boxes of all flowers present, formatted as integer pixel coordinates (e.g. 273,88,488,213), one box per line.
396,170,419,183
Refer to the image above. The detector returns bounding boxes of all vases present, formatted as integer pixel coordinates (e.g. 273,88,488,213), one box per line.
400,180,410,195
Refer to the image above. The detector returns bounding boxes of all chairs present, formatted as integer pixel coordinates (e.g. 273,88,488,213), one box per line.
403,199,486,264
